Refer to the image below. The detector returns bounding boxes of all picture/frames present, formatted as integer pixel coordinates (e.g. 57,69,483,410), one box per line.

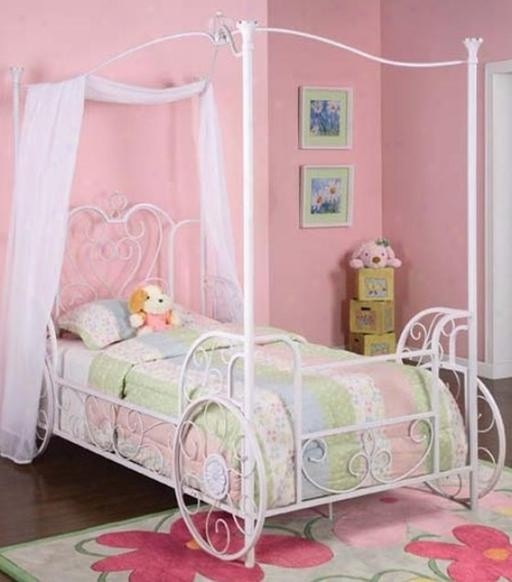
297,83,353,230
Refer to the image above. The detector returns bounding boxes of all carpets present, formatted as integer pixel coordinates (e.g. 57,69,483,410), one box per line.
1,459,512,582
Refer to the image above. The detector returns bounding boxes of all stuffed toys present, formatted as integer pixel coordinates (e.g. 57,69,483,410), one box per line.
348,238,402,271
126,281,185,336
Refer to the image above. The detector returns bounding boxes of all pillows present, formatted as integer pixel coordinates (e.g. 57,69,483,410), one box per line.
54,287,179,350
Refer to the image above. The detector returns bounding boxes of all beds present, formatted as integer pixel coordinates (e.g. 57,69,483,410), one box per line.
0,10,506,566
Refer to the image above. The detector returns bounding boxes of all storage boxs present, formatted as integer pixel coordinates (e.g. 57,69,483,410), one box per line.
348,268,396,355
